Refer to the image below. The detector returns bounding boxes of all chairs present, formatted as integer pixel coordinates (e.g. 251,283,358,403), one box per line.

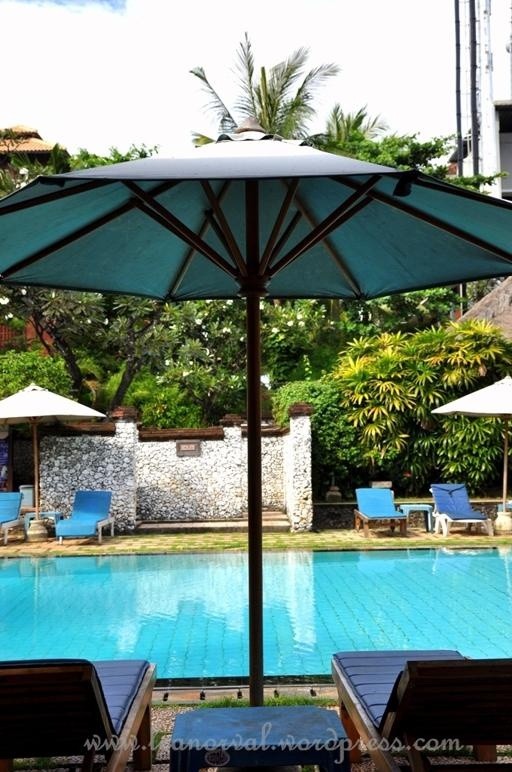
354,488,408,537
428,484,494,538
0,492,24,546
0,659,156,772
332,650,512,772
56,490,114,545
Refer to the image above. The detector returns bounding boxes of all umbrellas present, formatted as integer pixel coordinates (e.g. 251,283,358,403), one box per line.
1,127,511,700
1,384,106,518
430,375,511,511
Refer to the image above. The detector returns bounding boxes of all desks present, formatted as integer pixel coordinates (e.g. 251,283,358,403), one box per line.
399,504,433,533
170,706,350,772
25,512,64,532
